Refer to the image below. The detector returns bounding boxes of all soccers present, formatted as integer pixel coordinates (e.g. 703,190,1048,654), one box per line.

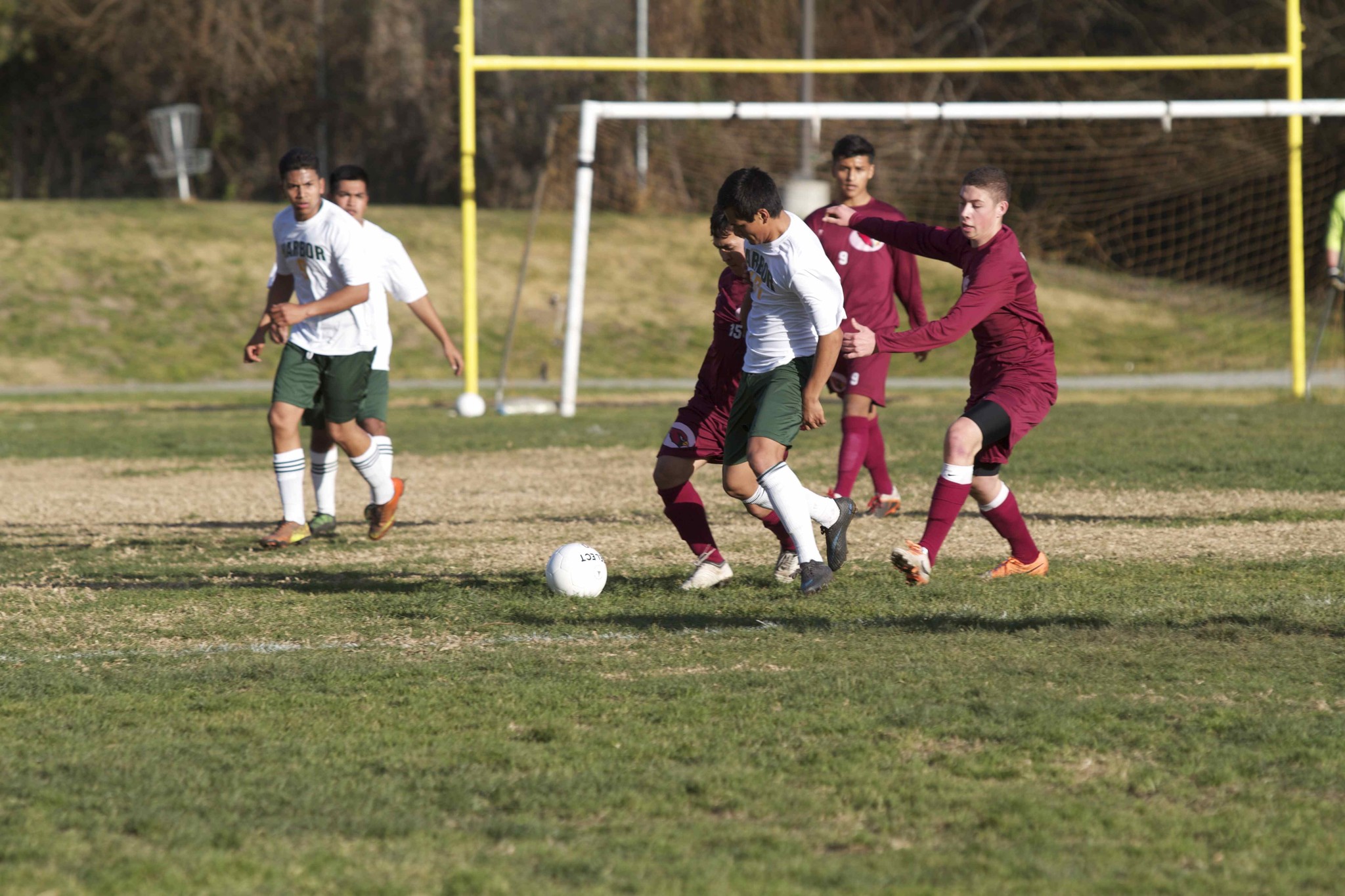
546,543,608,599
455,392,485,418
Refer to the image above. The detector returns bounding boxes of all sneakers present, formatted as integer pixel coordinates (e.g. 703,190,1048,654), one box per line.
890,546,932,585
797,560,833,596
978,552,1049,581
774,550,801,584
308,513,337,537
820,497,857,572
365,477,404,539
858,495,901,518
260,519,312,550
679,560,734,591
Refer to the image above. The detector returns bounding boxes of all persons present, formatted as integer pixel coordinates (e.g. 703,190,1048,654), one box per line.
238,145,409,547
259,165,468,542
822,166,1061,587
651,203,806,593
1323,188,1345,294
716,165,861,595
800,133,932,520
